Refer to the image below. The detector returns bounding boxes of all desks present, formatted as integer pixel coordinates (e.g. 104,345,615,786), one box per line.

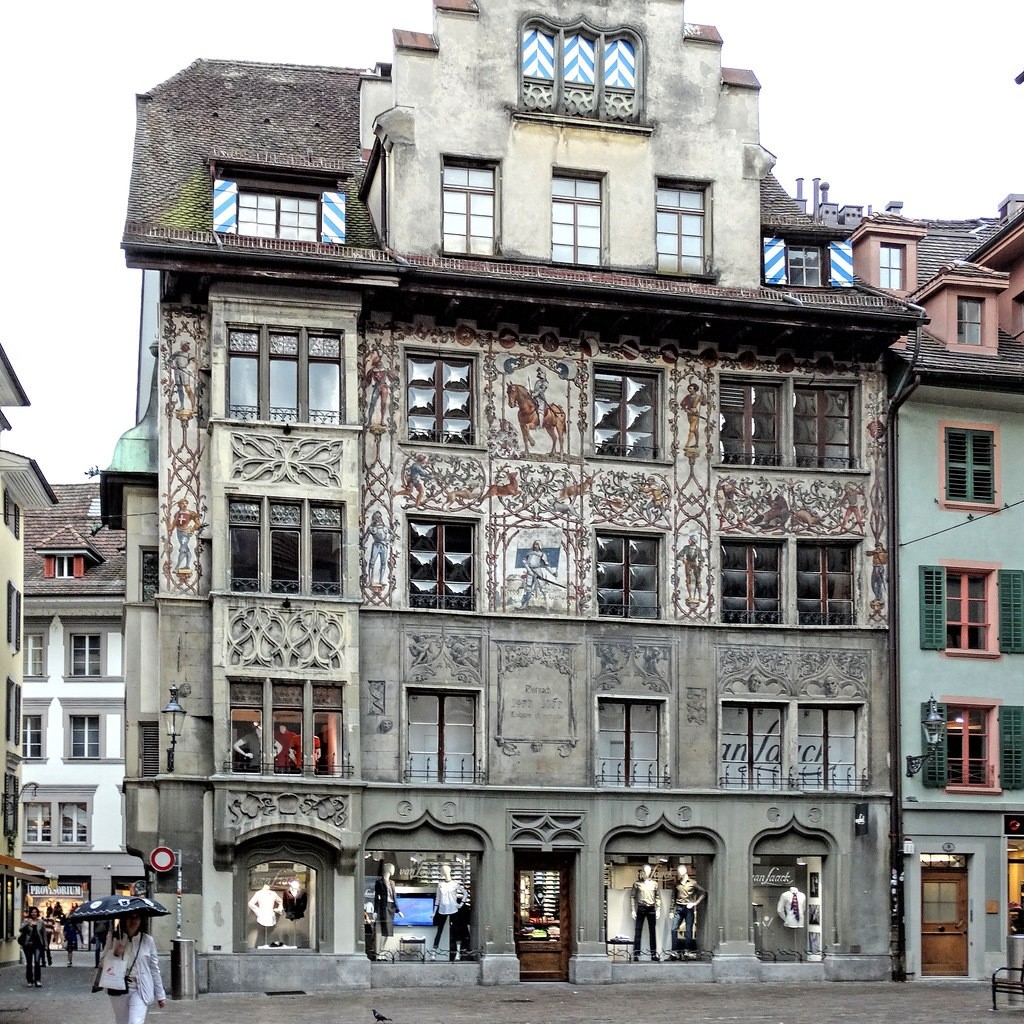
606,939,635,963
398,937,425,962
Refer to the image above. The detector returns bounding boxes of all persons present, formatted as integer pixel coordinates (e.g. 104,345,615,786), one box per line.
429,865,467,961
63,918,80,967
372,863,404,961
776,886,806,928
17,906,56,987
99,909,166,1024
247,879,309,927
94,932,102,968
233,722,322,774
53,901,86,949
629,865,708,961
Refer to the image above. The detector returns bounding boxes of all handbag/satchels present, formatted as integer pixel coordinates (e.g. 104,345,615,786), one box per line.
97,950,126,990
61,940,68,949
108,975,132,996
18,919,28,946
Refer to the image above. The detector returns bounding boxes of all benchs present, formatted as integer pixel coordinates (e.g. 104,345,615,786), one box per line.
991,960,1024,1010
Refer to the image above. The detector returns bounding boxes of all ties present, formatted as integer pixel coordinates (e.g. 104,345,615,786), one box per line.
792,893,799,921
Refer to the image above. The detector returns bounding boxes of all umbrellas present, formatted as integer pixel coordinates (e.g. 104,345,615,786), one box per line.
60,916,83,925
67,894,171,946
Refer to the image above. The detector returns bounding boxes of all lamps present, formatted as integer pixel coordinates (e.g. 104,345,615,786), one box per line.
906,691,946,778
160,683,188,772
365,851,467,864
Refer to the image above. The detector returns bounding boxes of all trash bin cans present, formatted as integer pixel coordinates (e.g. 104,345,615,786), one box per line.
1006,935,1024,1006
169,938,197,1000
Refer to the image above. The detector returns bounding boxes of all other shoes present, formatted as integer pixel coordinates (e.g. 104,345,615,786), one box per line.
40,964,46,967
48,957,52,965
634,957,638,961
664,957,676,961
652,956,659,961
67,961,72,967
28,983,33,987
35,981,41,986
